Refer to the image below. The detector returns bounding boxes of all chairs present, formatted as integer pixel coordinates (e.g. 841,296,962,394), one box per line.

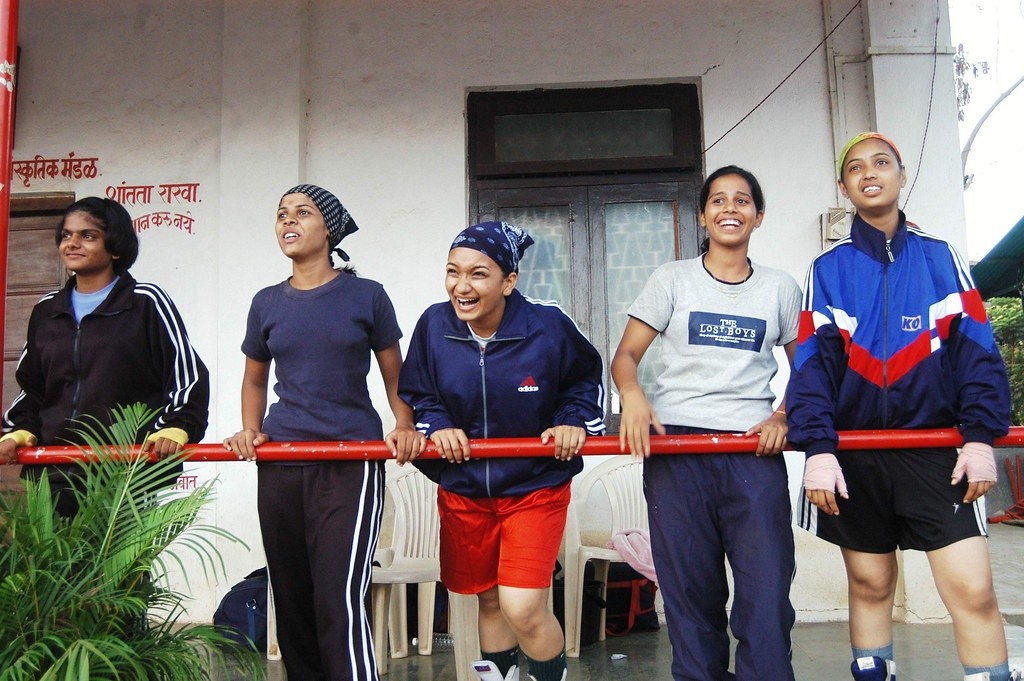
266,463,442,677
547,455,651,658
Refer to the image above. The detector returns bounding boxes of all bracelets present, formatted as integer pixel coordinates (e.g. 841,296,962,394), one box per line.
774,411,786,414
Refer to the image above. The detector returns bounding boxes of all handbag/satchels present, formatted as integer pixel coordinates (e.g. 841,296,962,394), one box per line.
364,581,448,639
555,560,660,636
213,567,268,653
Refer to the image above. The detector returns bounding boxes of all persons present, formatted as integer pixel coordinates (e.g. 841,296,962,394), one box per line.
397,220,606,681
0,197,209,640
786,132,1020,680
611,164,803,681
222,183,404,681
